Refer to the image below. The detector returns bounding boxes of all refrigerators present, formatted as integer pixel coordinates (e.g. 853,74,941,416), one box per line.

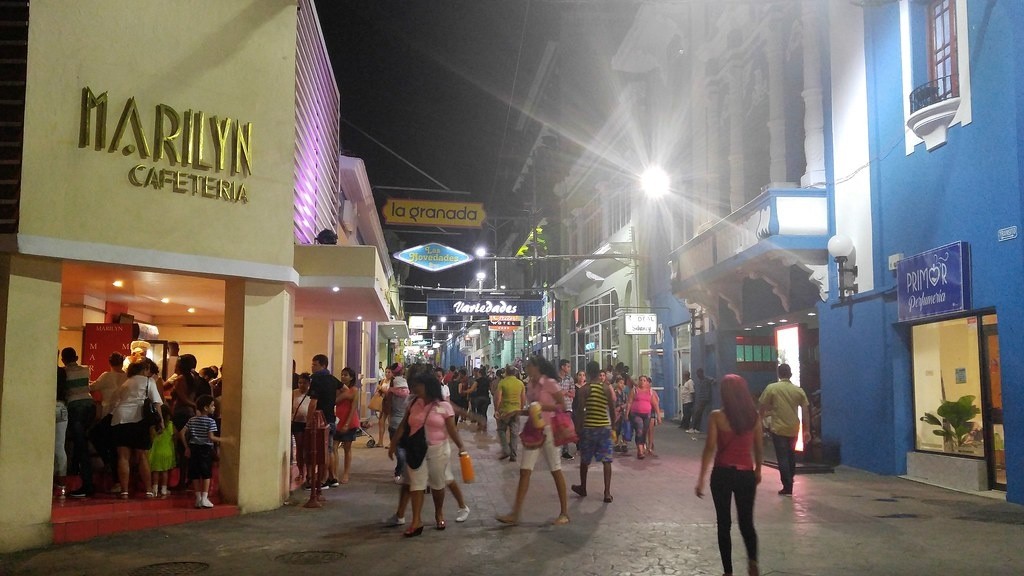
82,322,167,382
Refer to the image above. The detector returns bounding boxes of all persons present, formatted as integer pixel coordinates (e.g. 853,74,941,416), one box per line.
695,373,763,576
572,360,615,503
679,368,716,433
54,340,359,507
389,372,466,537
758,363,812,497
496,355,571,525
375,361,662,525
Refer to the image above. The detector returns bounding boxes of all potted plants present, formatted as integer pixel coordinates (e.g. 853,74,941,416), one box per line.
994,433,1005,464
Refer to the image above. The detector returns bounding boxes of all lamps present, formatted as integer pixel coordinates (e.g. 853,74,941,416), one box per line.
828,234,858,300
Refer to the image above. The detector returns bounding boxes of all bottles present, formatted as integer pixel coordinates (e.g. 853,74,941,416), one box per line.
529,402,546,428
462,452,475,484
610,430,617,441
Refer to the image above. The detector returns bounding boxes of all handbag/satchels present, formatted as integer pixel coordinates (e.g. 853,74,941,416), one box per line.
400,426,427,468
519,421,545,448
551,407,578,446
368,392,383,412
143,377,161,424
382,381,393,413
452,395,469,408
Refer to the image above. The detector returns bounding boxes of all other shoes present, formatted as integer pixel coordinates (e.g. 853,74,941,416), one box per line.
303,496,322,507
572,485,587,495
456,505,470,521
317,491,328,500
53,482,171,500
552,516,569,524
195,499,213,508
304,478,340,490
778,489,792,494
498,454,517,462
382,512,405,524
686,428,700,433
614,441,654,459
560,453,574,460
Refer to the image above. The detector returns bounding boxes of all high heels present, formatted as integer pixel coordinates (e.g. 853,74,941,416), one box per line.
404,523,423,536
459,418,466,422
437,520,445,529
